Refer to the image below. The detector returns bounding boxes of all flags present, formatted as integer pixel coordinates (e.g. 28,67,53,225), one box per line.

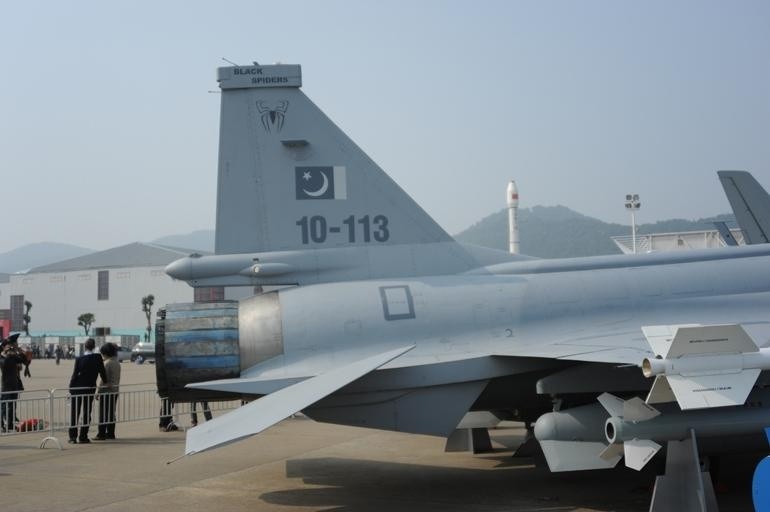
294,165,347,201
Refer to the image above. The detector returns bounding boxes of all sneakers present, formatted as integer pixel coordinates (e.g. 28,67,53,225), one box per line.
68,432,116,444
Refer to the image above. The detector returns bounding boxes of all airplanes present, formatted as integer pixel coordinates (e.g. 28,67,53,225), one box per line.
156,65,770,511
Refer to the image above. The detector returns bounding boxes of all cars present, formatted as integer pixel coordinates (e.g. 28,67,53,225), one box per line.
84,341,156,365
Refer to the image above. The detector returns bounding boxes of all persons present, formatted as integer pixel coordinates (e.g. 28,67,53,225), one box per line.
66,337,113,445
191,402,212,427
158,398,178,432
91,342,120,442
0,333,75,432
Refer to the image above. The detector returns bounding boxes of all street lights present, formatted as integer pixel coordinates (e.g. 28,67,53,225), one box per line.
623,193,641,255
